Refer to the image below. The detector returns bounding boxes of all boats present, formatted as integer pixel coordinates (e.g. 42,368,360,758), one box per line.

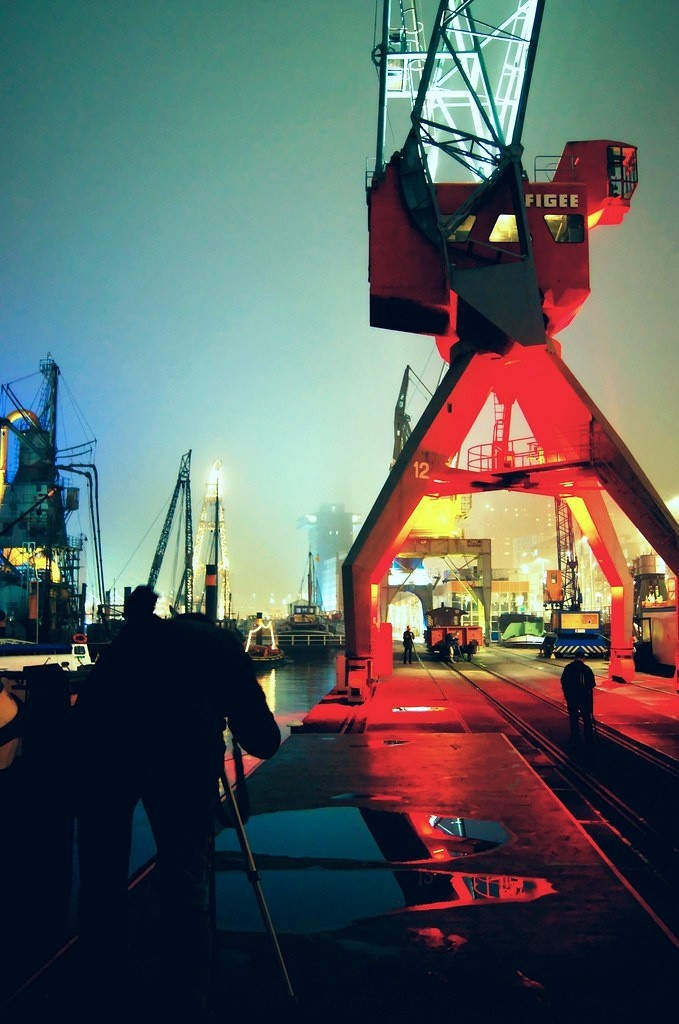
1,351,348,699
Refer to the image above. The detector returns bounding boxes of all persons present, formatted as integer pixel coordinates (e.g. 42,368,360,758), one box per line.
450,631,460,664
72,587,281,1023
403,626,415,664
561,651,596,755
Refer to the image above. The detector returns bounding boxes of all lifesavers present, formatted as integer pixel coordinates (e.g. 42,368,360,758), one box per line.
72,633,89,644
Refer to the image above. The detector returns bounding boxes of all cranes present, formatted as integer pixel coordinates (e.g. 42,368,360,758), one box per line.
540,492,611,659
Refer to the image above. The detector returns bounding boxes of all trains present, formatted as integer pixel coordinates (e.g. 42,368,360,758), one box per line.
424,602,482,662
